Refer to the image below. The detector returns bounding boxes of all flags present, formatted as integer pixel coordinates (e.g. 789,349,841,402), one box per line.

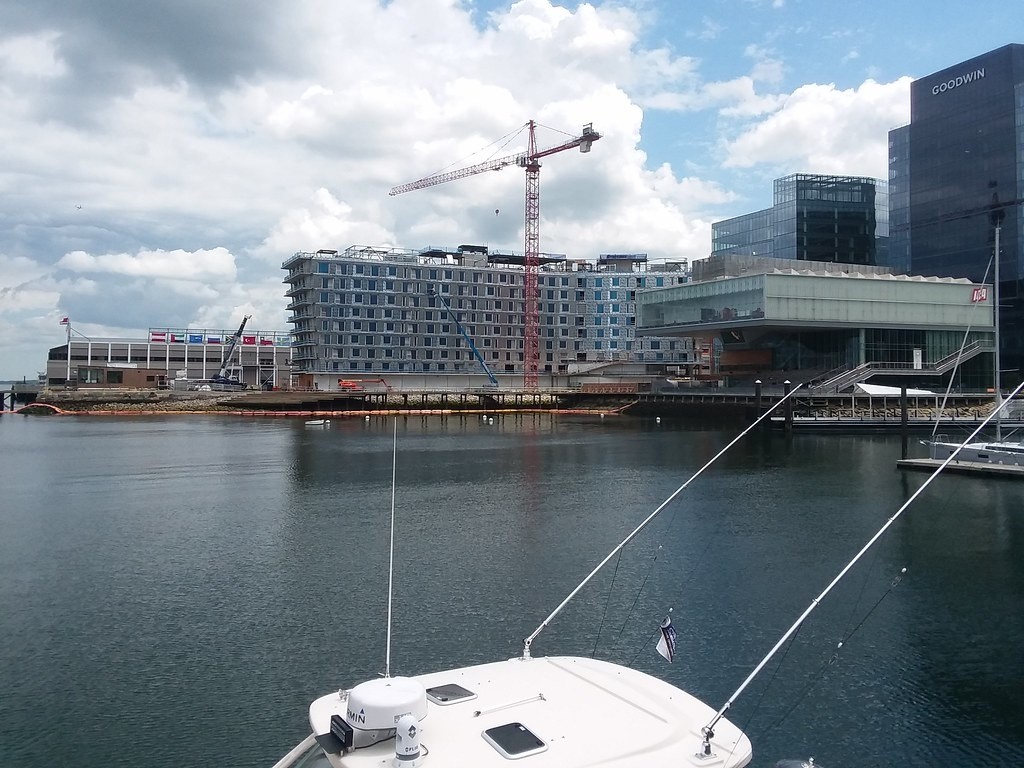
243,336,256,345
656,617,676,663
150,331,291,346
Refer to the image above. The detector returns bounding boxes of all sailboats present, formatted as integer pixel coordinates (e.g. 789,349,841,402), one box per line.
918,218,1024,467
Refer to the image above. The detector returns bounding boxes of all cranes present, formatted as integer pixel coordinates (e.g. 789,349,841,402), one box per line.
388,118,606,391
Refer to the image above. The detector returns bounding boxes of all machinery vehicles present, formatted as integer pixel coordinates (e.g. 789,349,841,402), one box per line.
210,314,253,390
337,377,395,392
